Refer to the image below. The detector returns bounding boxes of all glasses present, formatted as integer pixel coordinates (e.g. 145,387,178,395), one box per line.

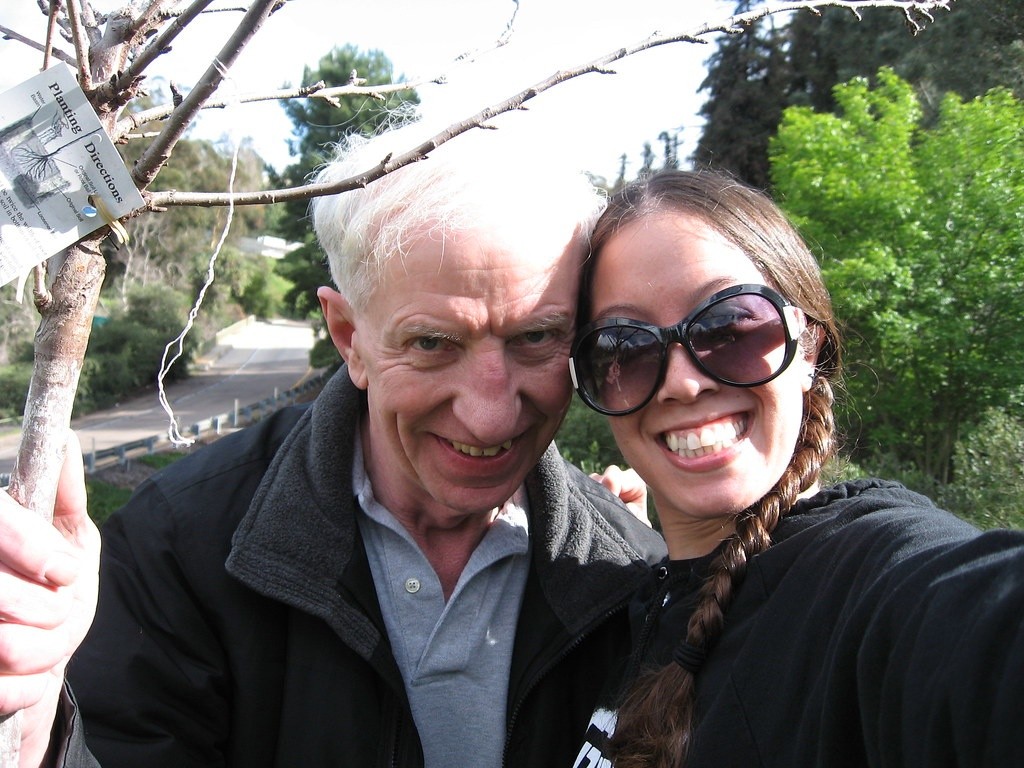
569,281,807,414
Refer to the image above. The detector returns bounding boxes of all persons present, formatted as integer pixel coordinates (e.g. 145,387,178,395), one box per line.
569,167,1024,768
0,106,669,768
